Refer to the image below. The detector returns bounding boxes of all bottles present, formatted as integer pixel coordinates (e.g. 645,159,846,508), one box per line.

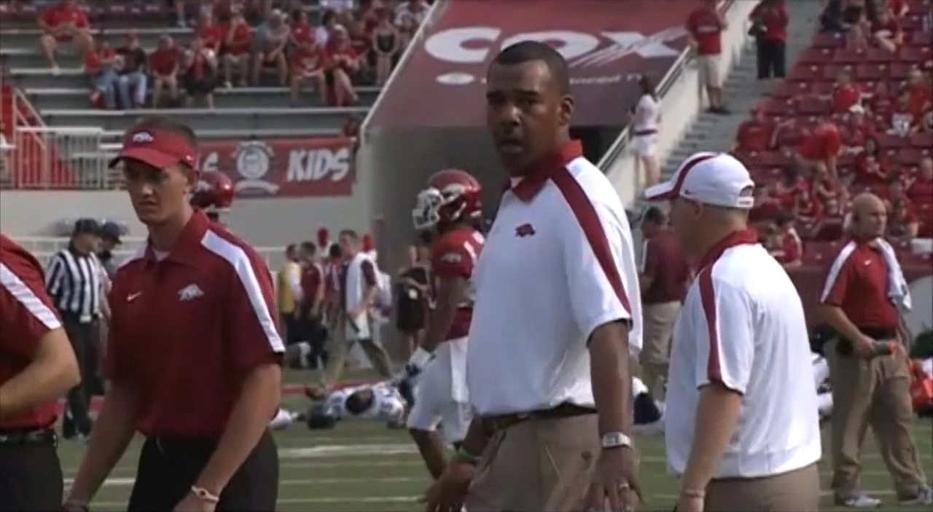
872,340,898,355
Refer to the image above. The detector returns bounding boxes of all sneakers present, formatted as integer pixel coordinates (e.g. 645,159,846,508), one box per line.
835,492,880,507
296,341,310,368
897,489,932,506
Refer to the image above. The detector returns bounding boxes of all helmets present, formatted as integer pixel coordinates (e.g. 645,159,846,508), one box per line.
192,171,233,209
419,169,482,222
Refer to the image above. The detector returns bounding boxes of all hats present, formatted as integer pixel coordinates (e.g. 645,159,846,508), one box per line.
108,126,197,171
76,219,105,242
645,152,755,209
103,223,121,245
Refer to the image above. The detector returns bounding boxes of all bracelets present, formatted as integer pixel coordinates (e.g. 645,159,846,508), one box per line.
678,487,708,497
456,445,486,466
191,484,222,505
407,343,433,374
65,497,88,511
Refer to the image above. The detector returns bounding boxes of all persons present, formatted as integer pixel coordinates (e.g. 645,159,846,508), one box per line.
412,168,487,479
814,191,933,508
624,77,665,189
0,232,82,510
43,218,112,441
643,150,822,512
814,0,932,60
91,219,128,394
65,118,287,511
638,204,691,404
100,220,130,280
685,1,730,115
415,43,647,512
192,169,431,433
1,0,425,134
748,1,791,79
734,60,933,270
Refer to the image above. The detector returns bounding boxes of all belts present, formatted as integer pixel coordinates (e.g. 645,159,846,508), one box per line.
0,429,56,444
483,402,596,434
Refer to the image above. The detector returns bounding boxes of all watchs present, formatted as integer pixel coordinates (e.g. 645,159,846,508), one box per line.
601,431,633,451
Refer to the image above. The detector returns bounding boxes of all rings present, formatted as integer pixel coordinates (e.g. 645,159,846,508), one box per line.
616,481,629,493
